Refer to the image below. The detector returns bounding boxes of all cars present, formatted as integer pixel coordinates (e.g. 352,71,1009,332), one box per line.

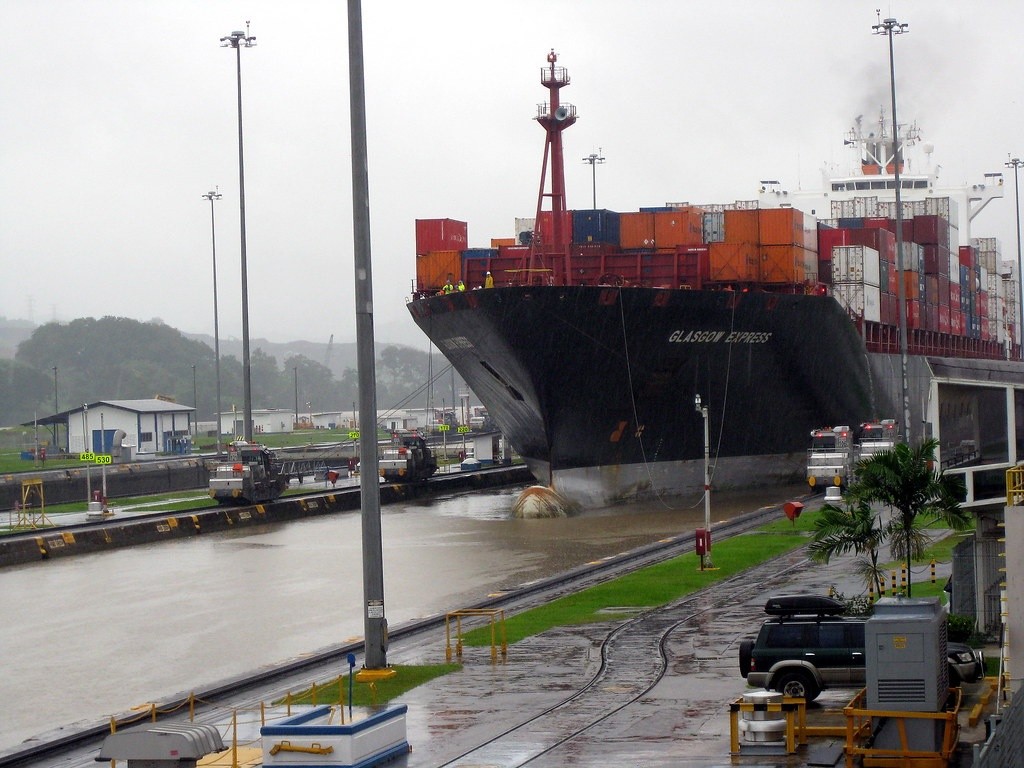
469,415,486,430
429,419,448,434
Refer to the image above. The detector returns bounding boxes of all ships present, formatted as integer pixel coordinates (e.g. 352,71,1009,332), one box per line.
408,55,1024,510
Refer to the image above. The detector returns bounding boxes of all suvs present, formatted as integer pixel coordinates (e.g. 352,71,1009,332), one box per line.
739,595,984,699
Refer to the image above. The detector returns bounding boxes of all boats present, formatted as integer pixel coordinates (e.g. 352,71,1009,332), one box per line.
207,438,291,504
380,428,438,481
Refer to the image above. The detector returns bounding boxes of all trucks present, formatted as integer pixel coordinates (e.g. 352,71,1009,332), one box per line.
859,418,902,462
806,426,858,490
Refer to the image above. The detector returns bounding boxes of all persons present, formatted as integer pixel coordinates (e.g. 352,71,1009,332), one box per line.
456,280,465,292
442,280,454,295
485,270,495,289
